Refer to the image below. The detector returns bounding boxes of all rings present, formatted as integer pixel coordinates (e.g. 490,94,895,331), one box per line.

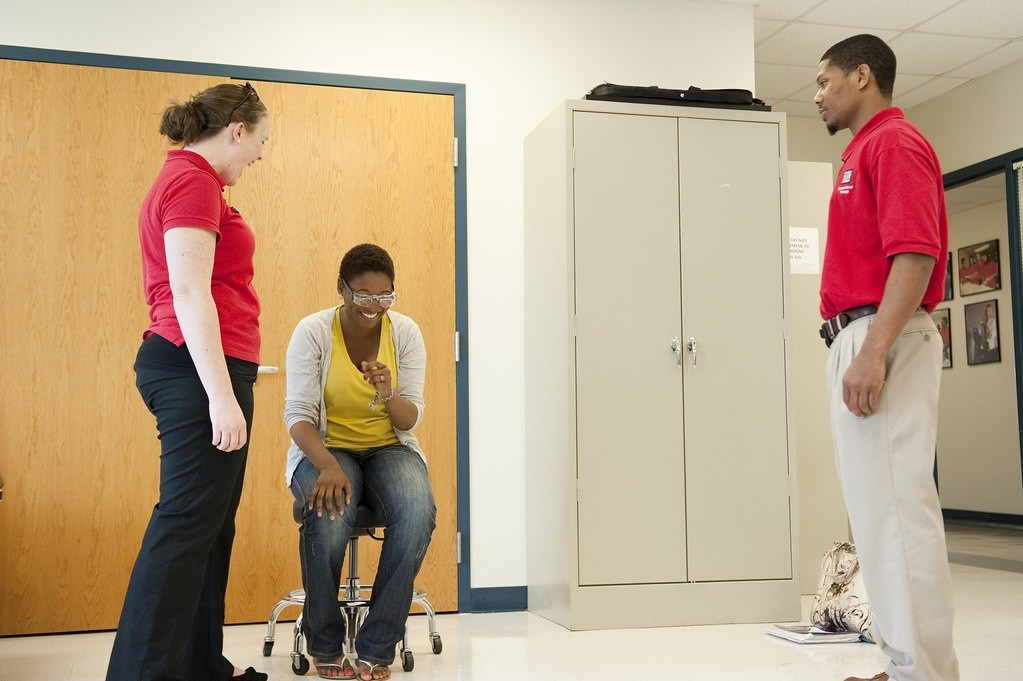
380,375,384,381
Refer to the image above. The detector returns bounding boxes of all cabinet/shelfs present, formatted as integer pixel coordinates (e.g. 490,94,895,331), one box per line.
787,160,849,596
523,99,802,632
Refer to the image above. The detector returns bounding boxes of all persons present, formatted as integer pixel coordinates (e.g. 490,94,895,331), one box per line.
939,316,949,361
978,304,998,362
959,254,999,289
286,244,438,681
813,33,960,681
106,83,270,681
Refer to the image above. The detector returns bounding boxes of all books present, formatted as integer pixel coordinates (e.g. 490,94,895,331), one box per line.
766,622,861,644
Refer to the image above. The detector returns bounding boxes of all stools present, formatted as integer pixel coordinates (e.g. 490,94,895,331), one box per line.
262,499,442,676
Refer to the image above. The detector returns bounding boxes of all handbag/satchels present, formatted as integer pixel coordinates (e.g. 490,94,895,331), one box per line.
808,541,875,643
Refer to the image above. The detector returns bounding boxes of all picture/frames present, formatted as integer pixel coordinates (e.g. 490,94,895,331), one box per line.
964,299,1001,365
929,308,953,369
943,252,953,301
957,239,1001,296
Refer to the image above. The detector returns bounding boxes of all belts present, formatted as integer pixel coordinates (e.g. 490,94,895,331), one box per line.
820,303,928,348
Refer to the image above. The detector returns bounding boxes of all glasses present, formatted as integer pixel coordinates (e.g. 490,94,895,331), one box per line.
340,278,397,308
225,81,260,127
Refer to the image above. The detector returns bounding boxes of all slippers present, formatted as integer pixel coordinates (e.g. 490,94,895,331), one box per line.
353,657,391,681
313,651,356,680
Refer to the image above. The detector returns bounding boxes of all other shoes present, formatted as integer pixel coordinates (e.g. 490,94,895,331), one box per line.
845,672,889,681
228,667,268,681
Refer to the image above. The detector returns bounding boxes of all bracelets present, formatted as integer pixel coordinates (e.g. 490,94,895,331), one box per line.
373,387,394,404
